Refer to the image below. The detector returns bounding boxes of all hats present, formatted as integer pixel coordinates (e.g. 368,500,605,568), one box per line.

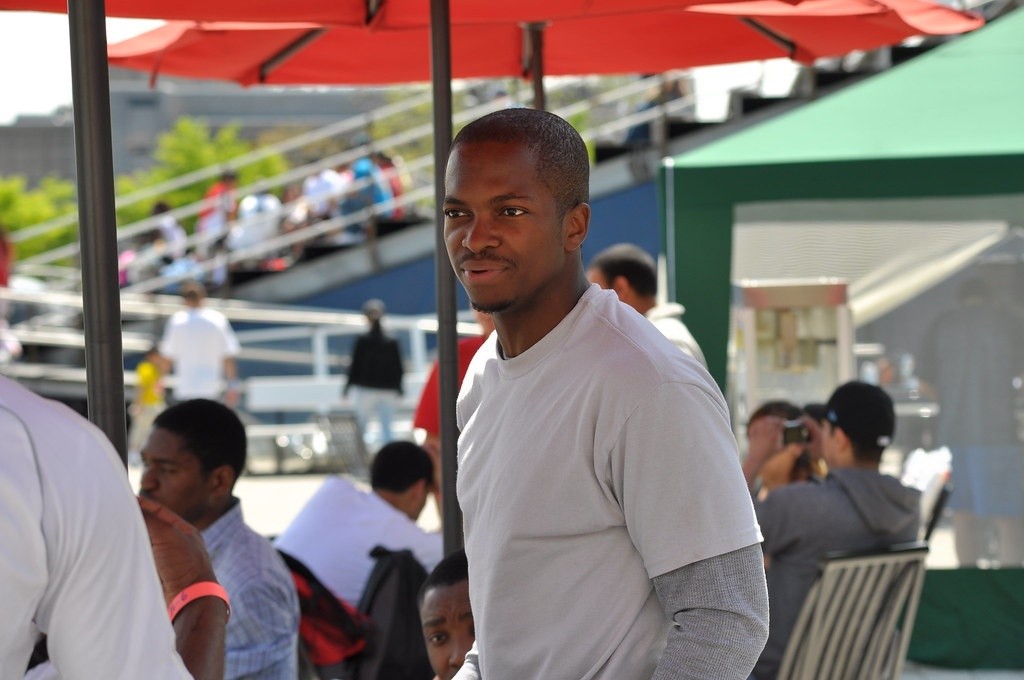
804,381,894,448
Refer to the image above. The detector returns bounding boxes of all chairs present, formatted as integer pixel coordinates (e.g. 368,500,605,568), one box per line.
777,545,928,679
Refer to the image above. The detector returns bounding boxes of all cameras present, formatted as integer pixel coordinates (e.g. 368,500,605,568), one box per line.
781,422,805,447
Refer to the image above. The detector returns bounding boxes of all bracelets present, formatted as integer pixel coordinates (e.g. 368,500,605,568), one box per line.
167,582,231,624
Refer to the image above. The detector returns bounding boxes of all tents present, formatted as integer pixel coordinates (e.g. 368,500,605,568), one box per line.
656,1,1023,674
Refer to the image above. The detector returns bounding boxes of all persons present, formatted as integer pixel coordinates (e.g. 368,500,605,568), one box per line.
125,348,173,463
159,285,240,406
0,229,193,680
743,382,922,680
343,301,405,442
275,440,445,607
442,106,770,680
139,398,300,680
414,309,496,441
416,548,475,680
587,245,711,367
124,149,407,294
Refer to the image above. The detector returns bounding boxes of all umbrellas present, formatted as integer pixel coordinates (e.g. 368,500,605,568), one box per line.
107,0,988,107
0,0,802,554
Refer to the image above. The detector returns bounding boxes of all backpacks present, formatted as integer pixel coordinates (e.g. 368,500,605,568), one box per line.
359,545,436,680
275,545,374,680
